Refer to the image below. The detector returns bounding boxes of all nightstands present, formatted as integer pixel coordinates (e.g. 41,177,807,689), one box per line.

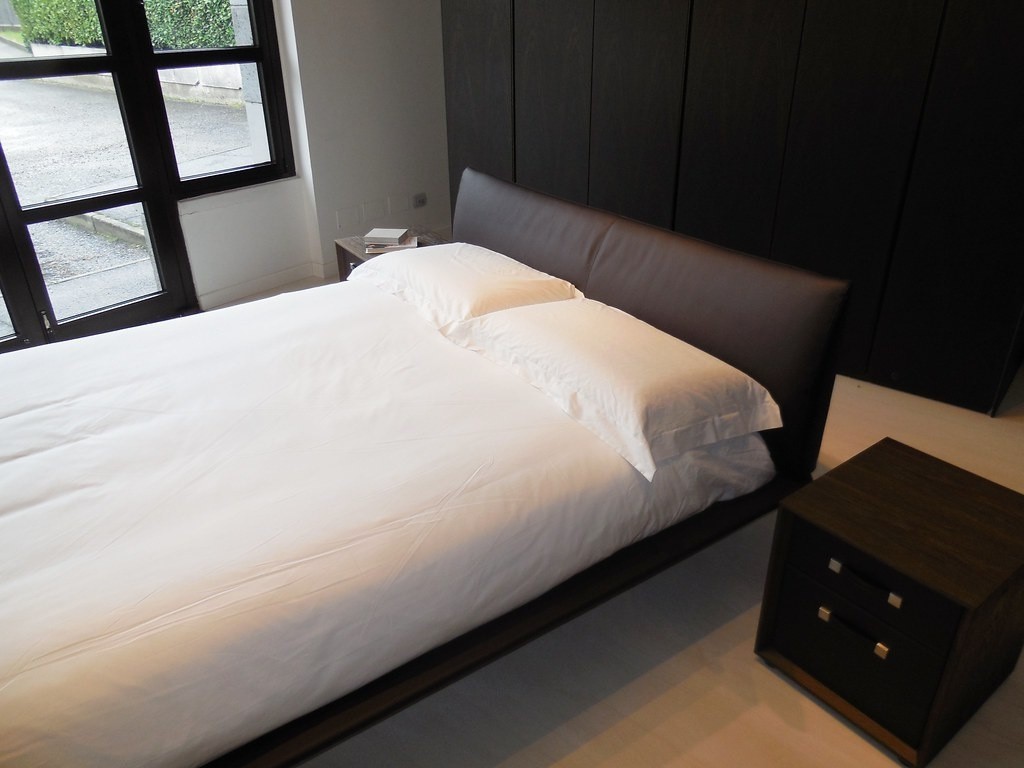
754,437,1024,768
333,224,451,281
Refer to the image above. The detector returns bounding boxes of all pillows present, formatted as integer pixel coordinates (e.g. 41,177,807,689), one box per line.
440,298,784,483
345,242,585,340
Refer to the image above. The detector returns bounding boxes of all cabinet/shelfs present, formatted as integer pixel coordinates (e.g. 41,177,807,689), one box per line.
441,0,1024,419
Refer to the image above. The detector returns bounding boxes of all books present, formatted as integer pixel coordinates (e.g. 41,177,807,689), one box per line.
365,236,418,254
363,227,409,245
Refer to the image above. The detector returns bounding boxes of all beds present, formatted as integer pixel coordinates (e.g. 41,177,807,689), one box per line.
0,169,855,768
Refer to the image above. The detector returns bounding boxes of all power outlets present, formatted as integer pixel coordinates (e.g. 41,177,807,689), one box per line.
413,192,426,207
